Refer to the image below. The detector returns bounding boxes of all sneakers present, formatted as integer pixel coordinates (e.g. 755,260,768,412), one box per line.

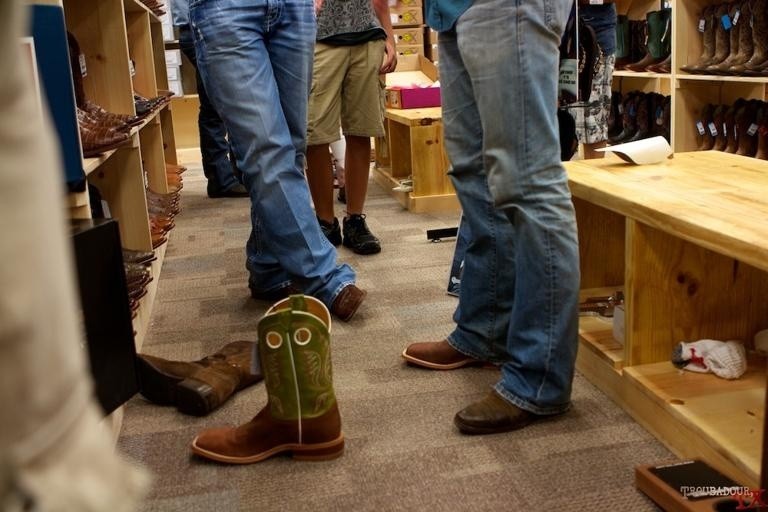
313,214,380,254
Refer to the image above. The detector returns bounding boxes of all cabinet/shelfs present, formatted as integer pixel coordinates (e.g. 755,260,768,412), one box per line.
371,105,464,214
578,1,768,159
25,0,179,450
560,148,767,499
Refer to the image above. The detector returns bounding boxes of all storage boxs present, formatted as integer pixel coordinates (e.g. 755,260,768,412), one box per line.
382,0,443,110
155,0,197,99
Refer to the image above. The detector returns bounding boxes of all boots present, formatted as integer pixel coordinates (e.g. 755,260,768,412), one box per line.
694,97,767,159
138,339,263,418
192,294,346,465
607,92,670,145
611,9,671,74
679,1,768,75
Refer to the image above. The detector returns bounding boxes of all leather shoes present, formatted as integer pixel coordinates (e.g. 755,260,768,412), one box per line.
402,339,477,371
209,183,250,197
142,162,188,249
77,89,174,154
118,248,158,320
332,283,367,322
454,390,544,436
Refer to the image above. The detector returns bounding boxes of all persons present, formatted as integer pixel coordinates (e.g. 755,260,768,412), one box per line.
187,1,368,321
401,0,581,436
305,0,398,255
167,0,246,198
563,0,616,159
2,0,154,508
333,122,351,202
